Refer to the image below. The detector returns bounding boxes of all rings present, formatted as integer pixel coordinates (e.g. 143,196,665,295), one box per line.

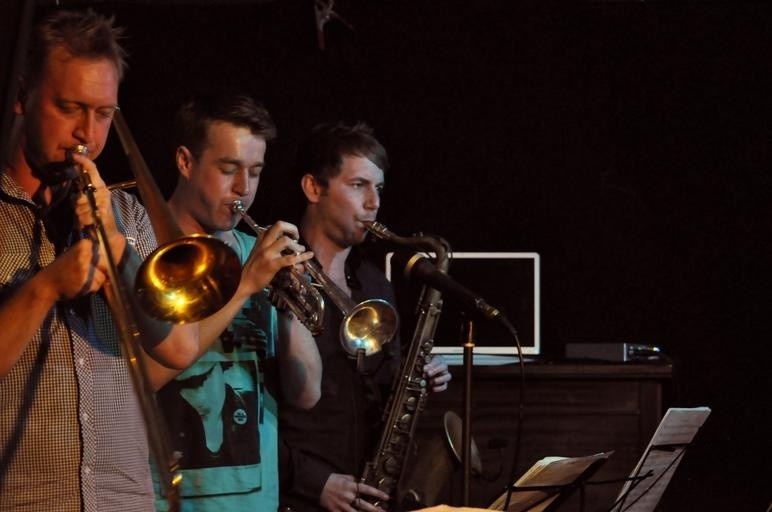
350,498,361,508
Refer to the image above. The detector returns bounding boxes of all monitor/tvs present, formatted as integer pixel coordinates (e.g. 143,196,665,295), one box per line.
384,250,543,367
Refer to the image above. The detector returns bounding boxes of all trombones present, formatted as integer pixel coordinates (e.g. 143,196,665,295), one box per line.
69,106,242,511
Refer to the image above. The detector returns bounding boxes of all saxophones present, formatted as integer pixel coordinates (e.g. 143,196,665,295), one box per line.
360,221,482,512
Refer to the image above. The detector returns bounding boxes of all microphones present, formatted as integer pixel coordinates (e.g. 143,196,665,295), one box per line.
313,4,328,53
389,246,518,335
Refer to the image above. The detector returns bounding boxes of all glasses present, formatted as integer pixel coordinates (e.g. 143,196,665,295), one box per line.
95,106,120,120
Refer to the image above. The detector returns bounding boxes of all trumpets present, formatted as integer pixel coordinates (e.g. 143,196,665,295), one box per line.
230,200,398,356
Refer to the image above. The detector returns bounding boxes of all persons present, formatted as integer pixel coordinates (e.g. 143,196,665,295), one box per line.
0,6,201,512
141,88,329,512
267,113,456,512
